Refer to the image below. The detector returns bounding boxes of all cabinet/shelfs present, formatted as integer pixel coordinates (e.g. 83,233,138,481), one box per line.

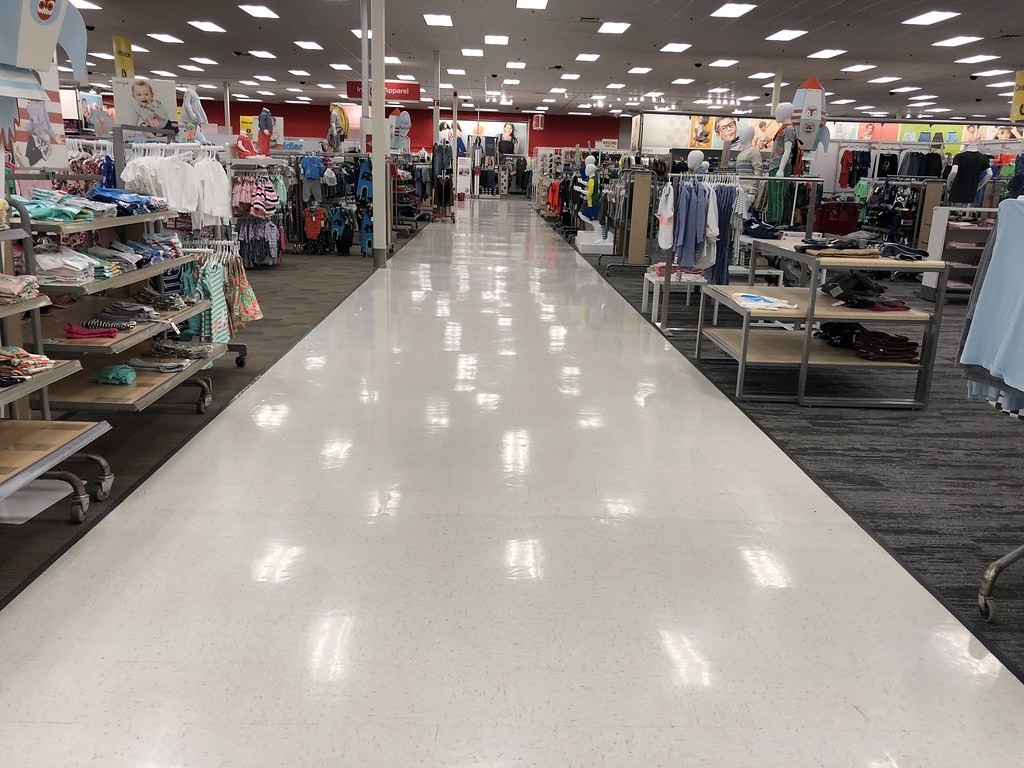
693,240,949,409
393,163,423,236
533,147,671,272
270,149,371,255
0,190,114,525
13,167,228,413
858,175,1000,304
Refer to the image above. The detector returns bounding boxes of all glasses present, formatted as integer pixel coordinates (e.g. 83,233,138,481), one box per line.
718,120,735,132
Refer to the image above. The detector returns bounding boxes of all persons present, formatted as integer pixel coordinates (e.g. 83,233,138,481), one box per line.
237,131,272,159
258,107,274,158
687,150,709,175
23,99,63,144
945,143,993,217
438,120,522,162
178,84,208,133
836,118,1024,146
573,155,606,244
81,98,103,128
735,126,764,226
328,108,344,153
691,116,772,152
764,102,798,236
131,80,179,139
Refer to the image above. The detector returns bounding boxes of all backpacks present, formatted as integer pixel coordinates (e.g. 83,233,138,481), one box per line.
793,147,804,175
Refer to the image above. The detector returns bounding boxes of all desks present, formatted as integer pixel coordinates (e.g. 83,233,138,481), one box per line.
642,273,721,325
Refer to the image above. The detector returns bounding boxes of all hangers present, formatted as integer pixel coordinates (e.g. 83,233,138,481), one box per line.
66,137,219,165
307,151,317,157
311,201,319,208
337,201,348,208
846,145,938,154
183,238,239,267
234,169,282,177
242,214,282,224
667,173,737,188
